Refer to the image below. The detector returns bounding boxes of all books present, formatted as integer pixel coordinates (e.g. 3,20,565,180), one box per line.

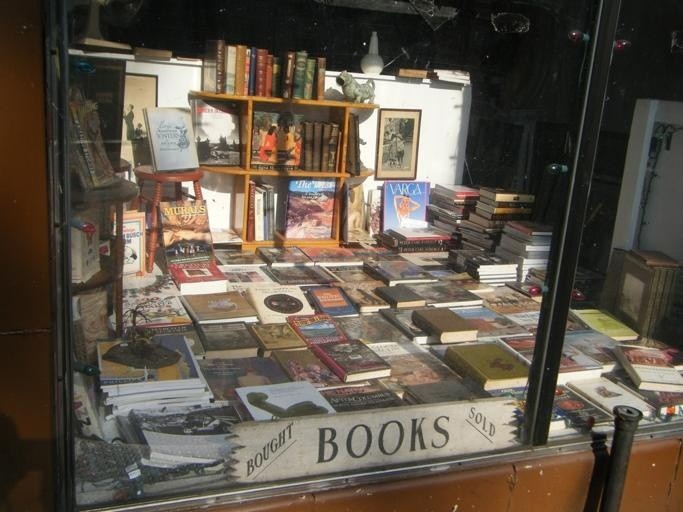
143,40,361,262
349,180,683,438
96,208,410,499
67,35,133,364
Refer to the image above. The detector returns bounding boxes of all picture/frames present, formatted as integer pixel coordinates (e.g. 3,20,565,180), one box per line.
124,72,158,166
378,108,423,180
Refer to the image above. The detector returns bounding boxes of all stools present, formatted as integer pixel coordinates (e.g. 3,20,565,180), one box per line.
136,166,206,272
65,183,140,341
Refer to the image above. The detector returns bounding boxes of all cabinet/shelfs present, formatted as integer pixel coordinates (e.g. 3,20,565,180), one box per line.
187,90,381,250
